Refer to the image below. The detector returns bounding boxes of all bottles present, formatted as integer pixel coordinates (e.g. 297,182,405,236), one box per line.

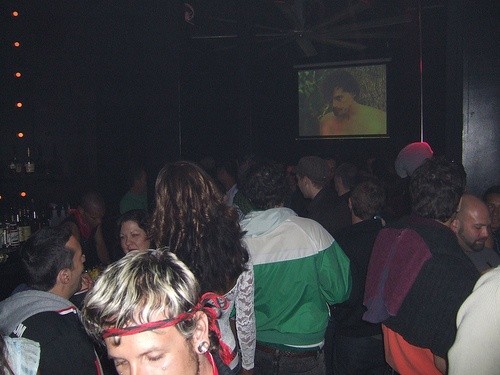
0,196,41,250
25,146,36,176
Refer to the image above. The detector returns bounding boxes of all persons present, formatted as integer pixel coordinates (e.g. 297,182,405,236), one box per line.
0,149,500,375
298,70,387,135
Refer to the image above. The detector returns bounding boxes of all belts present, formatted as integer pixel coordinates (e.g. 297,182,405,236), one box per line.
256,344,318,359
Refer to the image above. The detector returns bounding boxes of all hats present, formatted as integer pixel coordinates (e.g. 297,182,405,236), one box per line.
286,156,328,180
395,142,434,178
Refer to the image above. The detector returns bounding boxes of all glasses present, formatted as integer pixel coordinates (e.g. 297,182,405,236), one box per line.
72,254,85,263
488,205,500,212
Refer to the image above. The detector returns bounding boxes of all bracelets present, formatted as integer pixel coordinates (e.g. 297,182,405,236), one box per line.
84,271,88,273
